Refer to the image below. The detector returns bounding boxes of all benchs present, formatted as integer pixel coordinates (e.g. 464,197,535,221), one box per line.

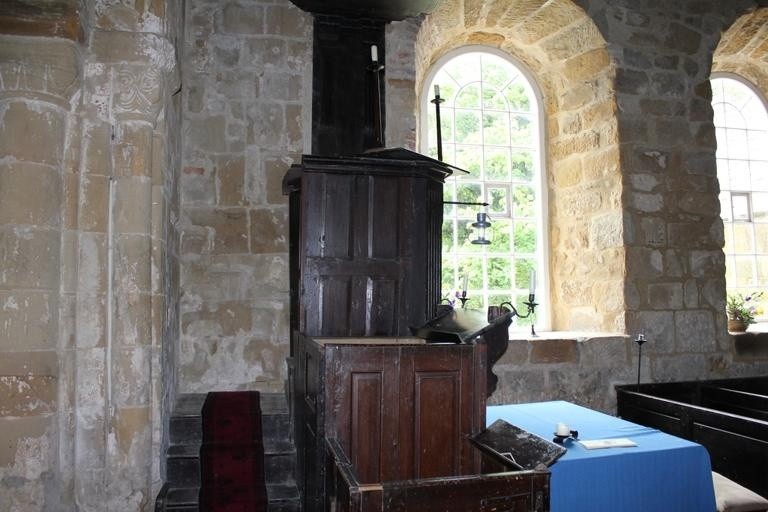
614,376,768,501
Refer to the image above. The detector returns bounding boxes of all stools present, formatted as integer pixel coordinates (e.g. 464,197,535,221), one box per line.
711,470,768,512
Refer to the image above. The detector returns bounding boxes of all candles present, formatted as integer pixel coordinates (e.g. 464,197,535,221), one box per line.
556,422,570,436
529,271,536,295
637,333,645,341
371,45,379,63
462,271,469,292
433,84,440,96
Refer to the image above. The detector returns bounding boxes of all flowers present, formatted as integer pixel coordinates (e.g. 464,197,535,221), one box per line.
726,291,765,324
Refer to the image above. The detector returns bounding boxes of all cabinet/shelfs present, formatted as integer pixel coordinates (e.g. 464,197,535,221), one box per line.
281,153,568,512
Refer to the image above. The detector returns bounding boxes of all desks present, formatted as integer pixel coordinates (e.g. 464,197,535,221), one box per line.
486,400,717,512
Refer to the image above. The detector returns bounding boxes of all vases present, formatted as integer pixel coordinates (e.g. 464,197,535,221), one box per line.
727,320,749,333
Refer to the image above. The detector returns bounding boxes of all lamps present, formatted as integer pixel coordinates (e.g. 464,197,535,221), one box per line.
467,204,495,245
